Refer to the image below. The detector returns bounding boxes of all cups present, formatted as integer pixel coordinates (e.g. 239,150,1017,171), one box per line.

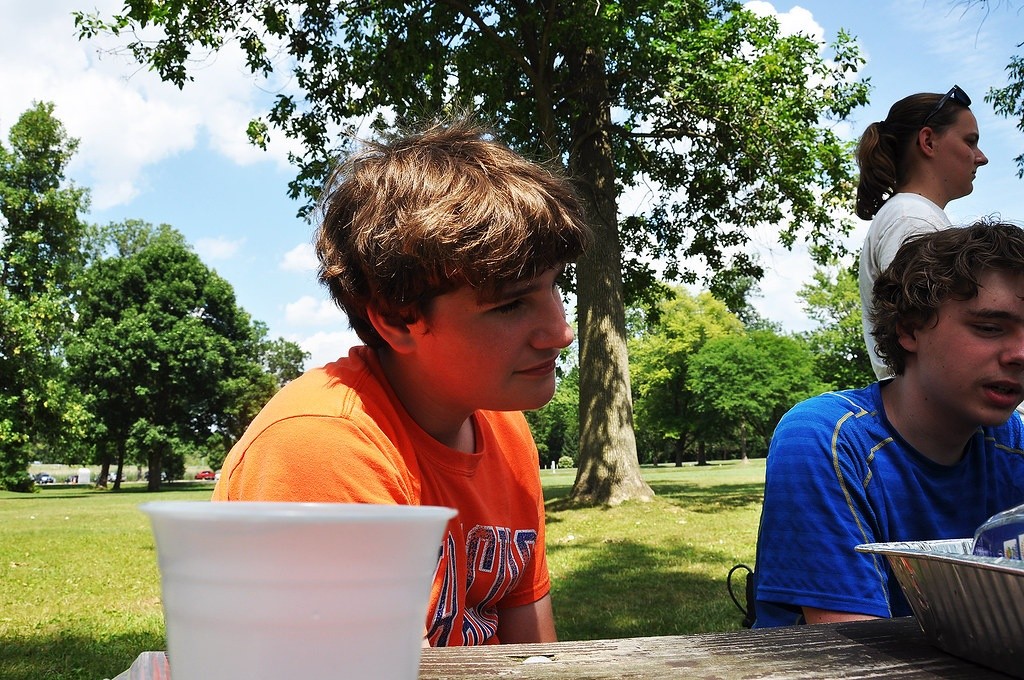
142,501,460,679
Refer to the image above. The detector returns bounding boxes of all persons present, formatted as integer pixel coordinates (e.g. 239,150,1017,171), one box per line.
856,90,989,385
209,121,577,647
747,214,1024,630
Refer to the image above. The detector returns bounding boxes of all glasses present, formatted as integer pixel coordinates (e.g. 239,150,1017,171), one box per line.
916,85,972,146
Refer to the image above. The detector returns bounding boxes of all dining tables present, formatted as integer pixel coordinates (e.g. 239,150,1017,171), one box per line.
98,613,1024,680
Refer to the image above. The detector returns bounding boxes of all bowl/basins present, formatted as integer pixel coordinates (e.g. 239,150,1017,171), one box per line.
854,536,1024,679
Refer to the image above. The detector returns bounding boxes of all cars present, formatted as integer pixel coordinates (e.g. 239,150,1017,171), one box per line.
144,469,170,481
94,471,128,481
35,472,55,484
65,475,79,482
195,471,216,480
